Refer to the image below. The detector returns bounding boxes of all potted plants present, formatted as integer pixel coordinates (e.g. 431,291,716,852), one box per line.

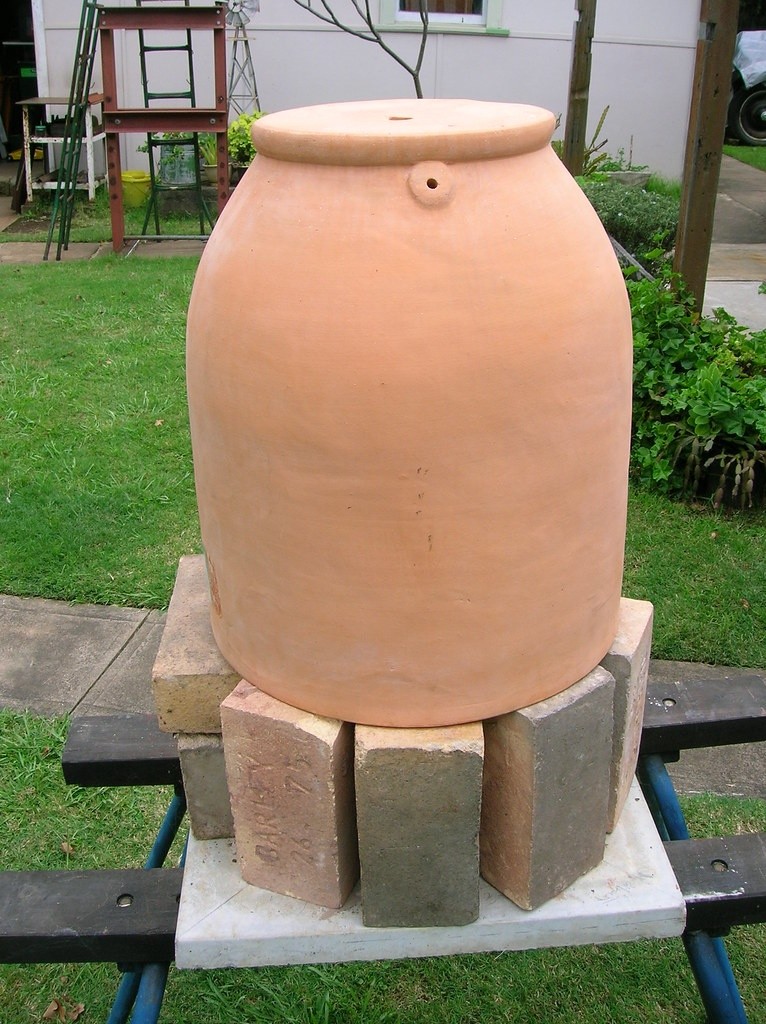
136,130,197,185
199,132,233,187
594,134,652,191
227,108,269,181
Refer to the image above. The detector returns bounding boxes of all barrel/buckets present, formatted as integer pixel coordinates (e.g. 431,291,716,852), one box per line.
187,97,634,731
121,169,151,208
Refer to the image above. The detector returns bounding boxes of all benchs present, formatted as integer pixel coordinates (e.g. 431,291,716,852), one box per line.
0,676,766,1024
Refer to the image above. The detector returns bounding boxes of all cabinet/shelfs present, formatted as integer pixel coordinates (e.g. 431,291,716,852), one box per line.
14,96,109,204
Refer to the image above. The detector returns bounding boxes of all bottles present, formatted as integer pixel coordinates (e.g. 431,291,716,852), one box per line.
35,125,46,137
